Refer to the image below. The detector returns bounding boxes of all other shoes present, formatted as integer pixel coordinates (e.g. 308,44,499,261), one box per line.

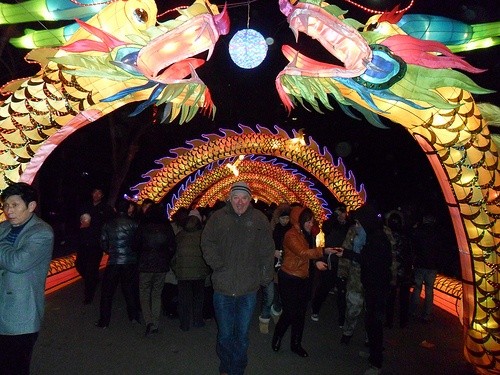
96,320,109,330
179,323,190,332
145,323,155,337
129,314,143,325
193,319,206,328
423,312,431,321
410,309,418,317
310,308,319,322
341,335,353,346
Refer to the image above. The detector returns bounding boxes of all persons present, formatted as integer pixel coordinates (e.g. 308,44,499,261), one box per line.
273,206,337,356
201,183,275,375
335,211,394,374
0,181,55,375
77,187,443,336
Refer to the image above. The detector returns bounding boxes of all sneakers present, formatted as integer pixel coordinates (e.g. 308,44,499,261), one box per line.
364,366,381,375
359,351,370,360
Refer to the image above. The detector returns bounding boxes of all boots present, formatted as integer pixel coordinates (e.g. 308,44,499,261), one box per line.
291,334,308,357
272,334,281,352
258,316,270,335
270,304,283,325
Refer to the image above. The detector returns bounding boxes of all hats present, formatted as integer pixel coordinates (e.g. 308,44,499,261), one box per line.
189,209,202,220
230,182,252,196
